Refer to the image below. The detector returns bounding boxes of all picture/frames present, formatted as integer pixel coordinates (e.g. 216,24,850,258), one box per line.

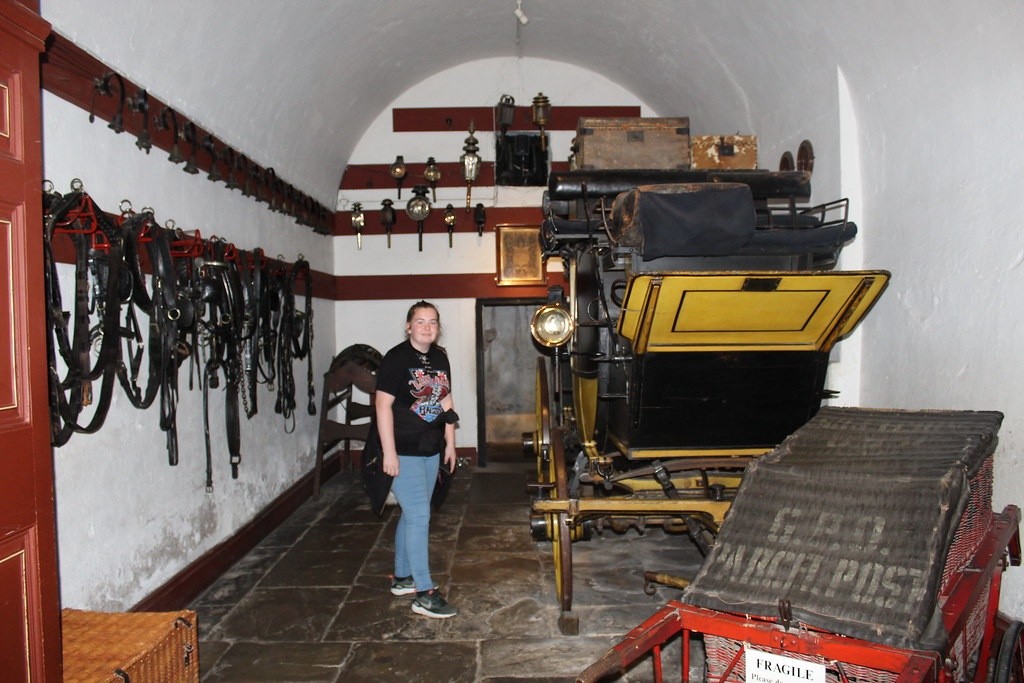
495,224,547,286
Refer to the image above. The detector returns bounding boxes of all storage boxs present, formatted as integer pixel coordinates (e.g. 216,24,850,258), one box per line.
58,608,199,683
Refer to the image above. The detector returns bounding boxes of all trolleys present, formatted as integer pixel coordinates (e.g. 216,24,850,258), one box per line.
572,407,1024,683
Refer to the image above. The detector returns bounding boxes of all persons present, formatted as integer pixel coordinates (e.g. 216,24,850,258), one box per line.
375,301,456,618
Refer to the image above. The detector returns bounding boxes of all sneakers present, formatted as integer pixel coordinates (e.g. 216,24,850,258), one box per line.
389,575,438,595
410,589,458,618
